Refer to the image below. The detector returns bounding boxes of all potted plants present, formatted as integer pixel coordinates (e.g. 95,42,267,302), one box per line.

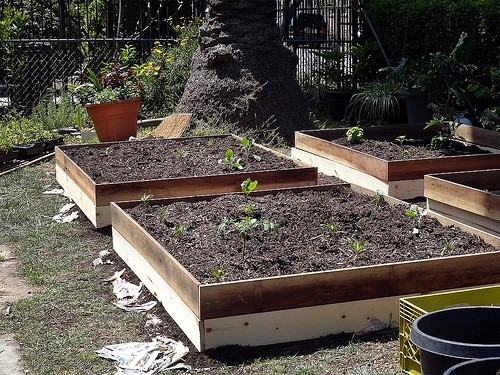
111,182,500,354
423,169,500,239
0,93,77,163
55,134,318,229
66,40,161,143
291,121,500,201
311,41,426,126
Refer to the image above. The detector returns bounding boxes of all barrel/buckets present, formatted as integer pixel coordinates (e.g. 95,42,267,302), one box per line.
443,357,500,375
411,306,500,375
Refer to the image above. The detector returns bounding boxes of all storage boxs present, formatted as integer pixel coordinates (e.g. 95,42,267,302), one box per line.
399,285,500,375
